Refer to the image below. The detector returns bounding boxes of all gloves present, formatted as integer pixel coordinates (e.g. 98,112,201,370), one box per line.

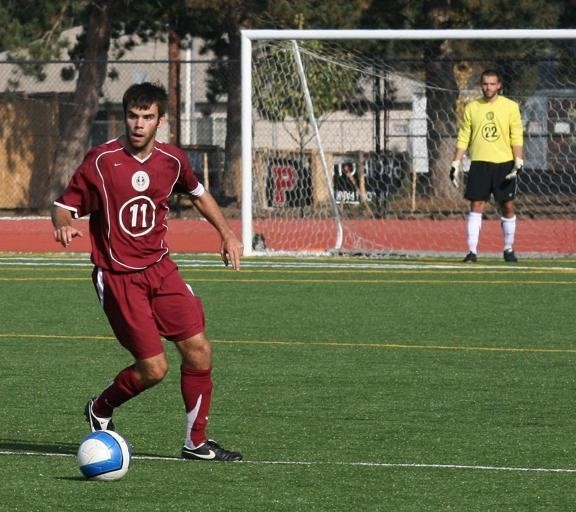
504,157,525,179
450,159,461,189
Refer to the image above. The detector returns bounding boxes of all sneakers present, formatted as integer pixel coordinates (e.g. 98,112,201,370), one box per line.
461,252,477,264
83,397,115,433
181,438,243,461
504,247,518,262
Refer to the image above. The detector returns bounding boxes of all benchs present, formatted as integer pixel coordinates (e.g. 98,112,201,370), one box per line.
387,193,576,219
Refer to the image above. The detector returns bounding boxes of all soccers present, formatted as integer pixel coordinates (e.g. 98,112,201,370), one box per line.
78,430,129,482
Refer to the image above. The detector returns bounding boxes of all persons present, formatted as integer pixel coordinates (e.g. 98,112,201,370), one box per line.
337,163,354,190
47,79,245,462
449,68,525,263
